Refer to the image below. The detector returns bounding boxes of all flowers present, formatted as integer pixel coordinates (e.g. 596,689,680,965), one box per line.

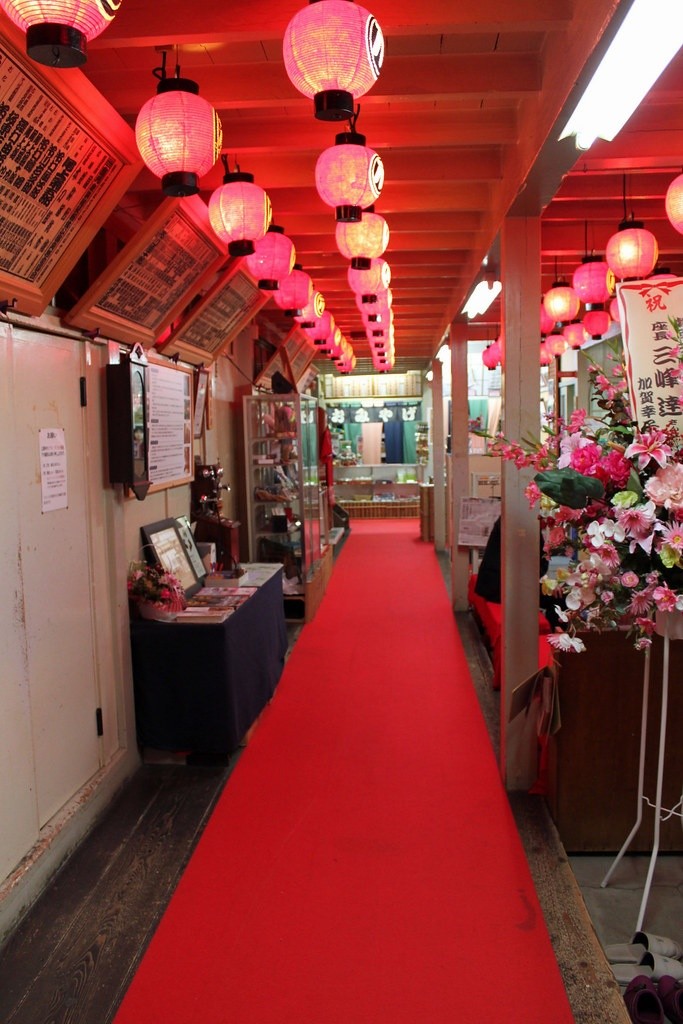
469,316,683,654
126,559,188,613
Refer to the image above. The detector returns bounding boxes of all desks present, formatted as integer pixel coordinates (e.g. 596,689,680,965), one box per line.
549,631,683,851
129,562,289,758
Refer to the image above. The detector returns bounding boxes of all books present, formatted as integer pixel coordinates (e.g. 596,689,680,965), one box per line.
176,571,258,624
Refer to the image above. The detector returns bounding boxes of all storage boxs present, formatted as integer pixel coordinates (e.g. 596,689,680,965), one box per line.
196,542,217,574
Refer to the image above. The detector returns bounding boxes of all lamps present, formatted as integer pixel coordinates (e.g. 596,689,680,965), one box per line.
460,271,503,318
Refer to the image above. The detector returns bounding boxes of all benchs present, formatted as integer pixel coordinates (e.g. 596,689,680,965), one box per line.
467,563,551,690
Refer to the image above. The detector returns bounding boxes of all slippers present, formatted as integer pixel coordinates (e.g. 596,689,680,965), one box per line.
603,931,678,963
610,951,683,986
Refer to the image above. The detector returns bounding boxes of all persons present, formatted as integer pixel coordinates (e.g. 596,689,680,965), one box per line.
133,426,145,459
337,446,357,465
133,396,143,424
474,513,572,634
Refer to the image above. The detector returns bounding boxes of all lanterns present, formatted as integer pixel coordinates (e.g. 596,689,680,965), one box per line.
294,290,335,345
315,132,385,223
315,325,356,375
362,308,395,374
0,0,122,68
283,0,384,121
246,225,313,317
208,172,272,256
665,173,683,235
540,255,620,367
348,258,392,322
605,221,658,282
135,78,223,197
482,335,502,370
335,205,389,270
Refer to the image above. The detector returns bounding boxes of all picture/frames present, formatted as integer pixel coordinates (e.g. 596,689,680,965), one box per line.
140,517,202,598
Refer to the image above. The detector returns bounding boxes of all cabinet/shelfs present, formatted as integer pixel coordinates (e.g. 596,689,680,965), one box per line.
331,463,424,519
242,393,333,625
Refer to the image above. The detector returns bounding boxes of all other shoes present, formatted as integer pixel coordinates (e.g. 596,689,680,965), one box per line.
623,974,665,1024
657,975,683,1024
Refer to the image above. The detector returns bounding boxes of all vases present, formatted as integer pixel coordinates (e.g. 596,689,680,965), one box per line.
140,605,171,619
